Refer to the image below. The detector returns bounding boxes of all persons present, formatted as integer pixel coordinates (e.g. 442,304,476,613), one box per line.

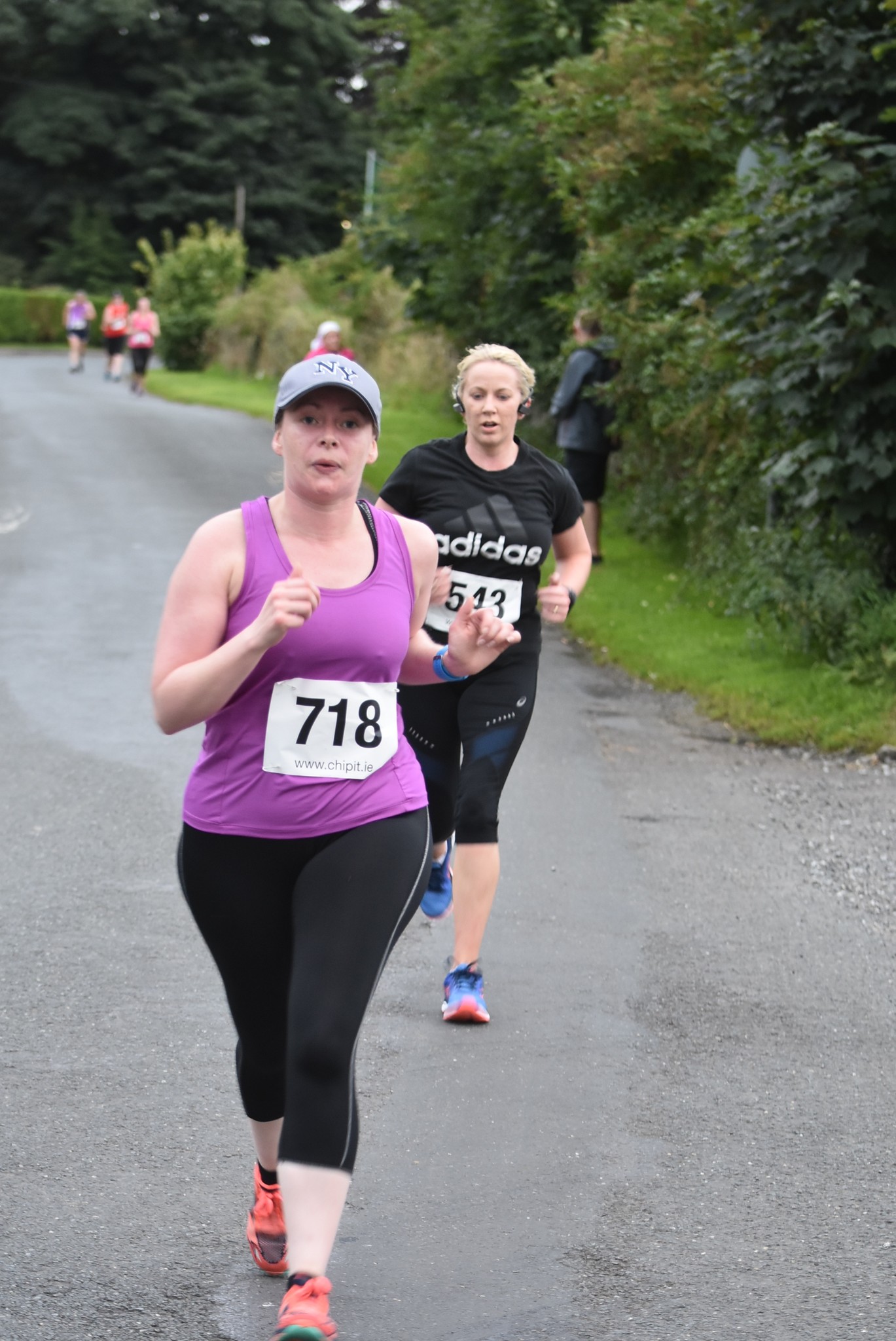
374,344,594,1024
546,310,628,563
305,321,356,363
99,290,162,391
62,290,97,369
149,353,522,1341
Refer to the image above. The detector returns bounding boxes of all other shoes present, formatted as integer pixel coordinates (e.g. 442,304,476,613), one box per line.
592,556,601,563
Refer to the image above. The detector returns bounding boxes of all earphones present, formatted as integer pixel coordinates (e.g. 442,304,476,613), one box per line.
452,395,465,413
518,398,529,414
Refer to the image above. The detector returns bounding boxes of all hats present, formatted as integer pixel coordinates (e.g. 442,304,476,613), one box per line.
274,354,382,442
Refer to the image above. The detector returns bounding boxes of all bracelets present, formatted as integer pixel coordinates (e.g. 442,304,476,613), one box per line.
433,644,468,681
564,585,576,615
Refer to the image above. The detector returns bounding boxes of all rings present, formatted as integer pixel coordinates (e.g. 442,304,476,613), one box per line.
553,605,559,612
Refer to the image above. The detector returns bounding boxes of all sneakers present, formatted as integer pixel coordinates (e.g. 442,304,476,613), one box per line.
246,1163,289,1277
269,1276,338,1341
441,958,490,1023
420,830,456,921
